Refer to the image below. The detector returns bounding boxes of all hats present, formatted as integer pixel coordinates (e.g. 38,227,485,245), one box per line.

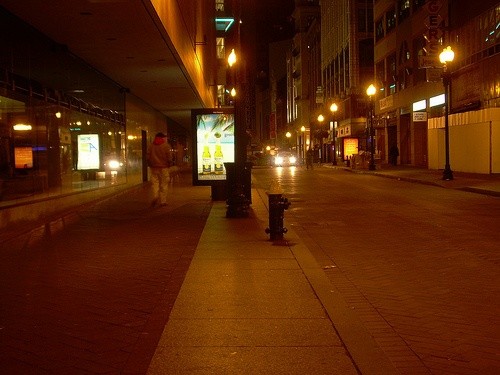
156,132,166,137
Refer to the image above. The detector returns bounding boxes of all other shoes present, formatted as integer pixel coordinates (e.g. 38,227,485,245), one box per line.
153,197,167,206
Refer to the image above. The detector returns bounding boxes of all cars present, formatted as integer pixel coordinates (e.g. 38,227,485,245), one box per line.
275,151,296,166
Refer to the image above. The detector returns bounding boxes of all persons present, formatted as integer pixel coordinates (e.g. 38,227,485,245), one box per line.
390,144,399,166
147,133,169,205
305,147,314,169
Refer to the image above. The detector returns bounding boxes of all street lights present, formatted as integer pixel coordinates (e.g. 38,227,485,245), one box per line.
330,102,337,166
317,114,325,163
366,83,377,170
285,131,291,145
300,125,306,164
438,46,454,180
